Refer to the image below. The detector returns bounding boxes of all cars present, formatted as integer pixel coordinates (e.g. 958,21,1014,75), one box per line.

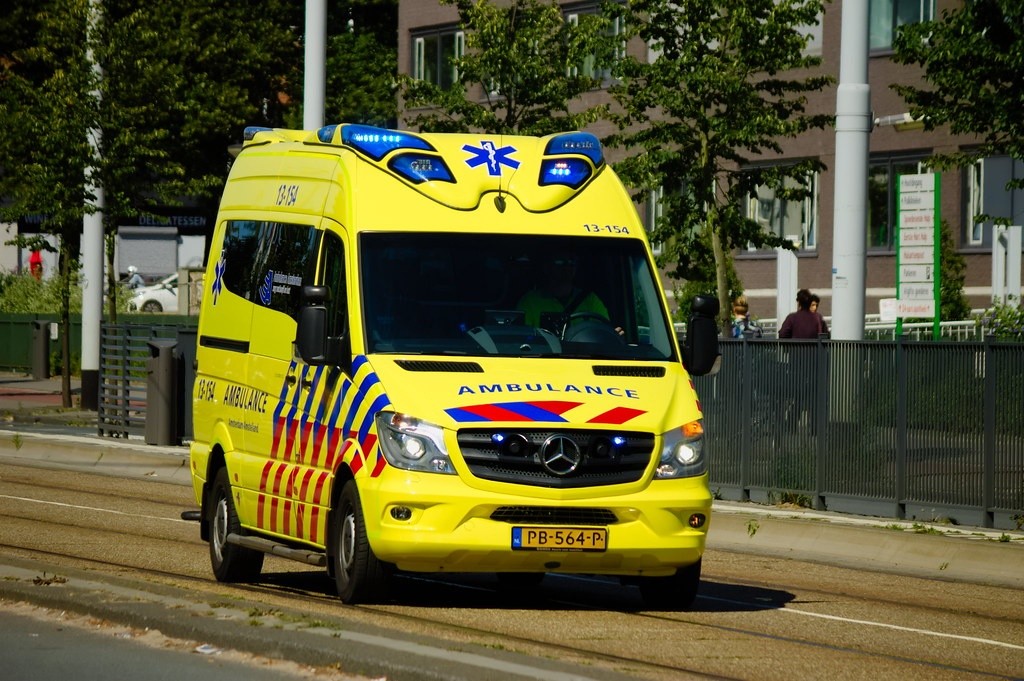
127,271,181,316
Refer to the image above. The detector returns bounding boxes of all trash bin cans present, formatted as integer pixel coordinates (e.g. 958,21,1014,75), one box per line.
145,336,181,444
30,319,51,380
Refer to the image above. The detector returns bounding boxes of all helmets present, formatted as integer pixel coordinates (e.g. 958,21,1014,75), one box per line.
127,265,137,274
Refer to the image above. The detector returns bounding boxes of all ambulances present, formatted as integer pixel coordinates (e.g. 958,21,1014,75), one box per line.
182,121,721,611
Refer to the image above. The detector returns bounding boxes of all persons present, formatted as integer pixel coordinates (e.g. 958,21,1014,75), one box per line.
517,246,626,336
28,251,42,281
121,265,144,289
716,288,829,436
